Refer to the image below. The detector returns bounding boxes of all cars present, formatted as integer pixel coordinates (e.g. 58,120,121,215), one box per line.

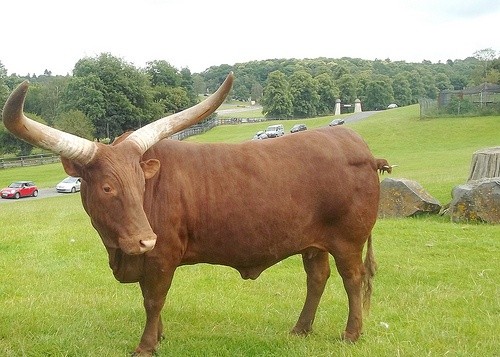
290,124,307,133
329,119,344,127
0,181,38,199
387,103,398,108
56,176,83,193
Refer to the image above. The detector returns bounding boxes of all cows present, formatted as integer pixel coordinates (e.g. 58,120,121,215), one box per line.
376,159,392,175
2,70,380,357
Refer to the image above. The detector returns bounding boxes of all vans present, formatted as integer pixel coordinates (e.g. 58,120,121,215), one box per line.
265,125,284,138
